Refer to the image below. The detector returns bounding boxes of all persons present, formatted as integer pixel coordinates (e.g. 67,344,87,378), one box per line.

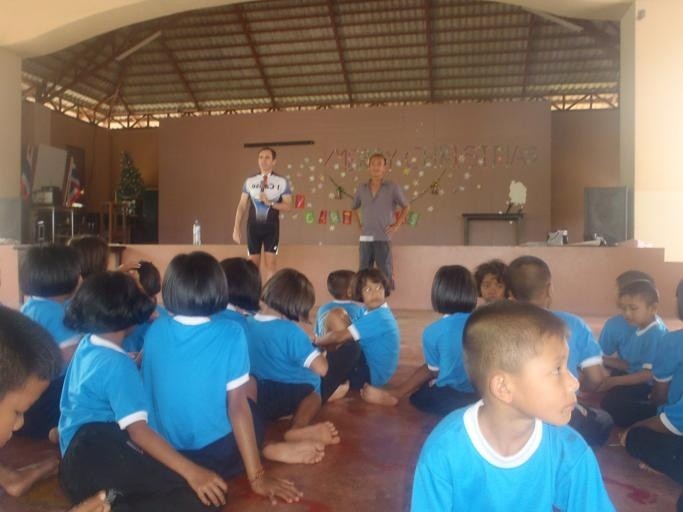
351,154,410,296
313,269,399,401
116,260,161,354
594,280,670,422
142,251,303,505
407,263,478,415
210,257,340,464
233,147,294,279
14,242,81,443
616,398,683,482
68,235,115,277
411,302,617,512
1,304,55,444
246,265,358,401
59,271,228,511
509,256,611,452
317,271,363,332
596,270,654,352
614,281,683,426
475,260,509,305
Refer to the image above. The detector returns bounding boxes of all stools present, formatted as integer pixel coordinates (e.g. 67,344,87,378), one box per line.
100,202,131,245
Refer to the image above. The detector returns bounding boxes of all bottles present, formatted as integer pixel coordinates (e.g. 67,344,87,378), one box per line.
192,219,200,246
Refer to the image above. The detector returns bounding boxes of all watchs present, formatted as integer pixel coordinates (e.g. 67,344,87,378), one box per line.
269,201,274,206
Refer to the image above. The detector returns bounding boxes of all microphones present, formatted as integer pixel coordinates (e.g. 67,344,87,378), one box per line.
259,175,268,204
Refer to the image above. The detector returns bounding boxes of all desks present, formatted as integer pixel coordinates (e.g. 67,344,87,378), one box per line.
460,212,526,246
32,205,76,246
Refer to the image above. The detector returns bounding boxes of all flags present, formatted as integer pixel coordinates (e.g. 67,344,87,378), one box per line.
20,147,32,204
64,148,84,209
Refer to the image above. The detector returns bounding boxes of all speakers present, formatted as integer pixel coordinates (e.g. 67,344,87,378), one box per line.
582,186,628,246
